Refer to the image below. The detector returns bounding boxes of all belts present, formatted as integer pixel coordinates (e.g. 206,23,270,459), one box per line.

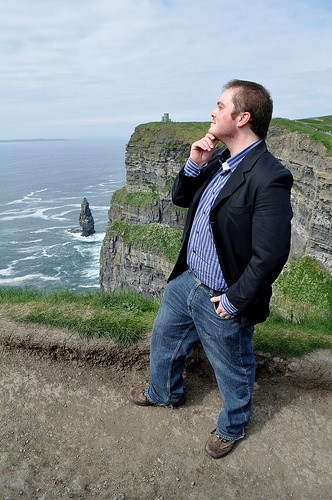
194,276,226,295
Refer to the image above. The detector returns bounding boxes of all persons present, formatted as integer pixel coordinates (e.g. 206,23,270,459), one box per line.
127,78,293,458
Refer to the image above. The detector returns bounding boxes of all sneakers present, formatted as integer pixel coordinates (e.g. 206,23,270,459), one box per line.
126,385,188,408
205,429,235,459
